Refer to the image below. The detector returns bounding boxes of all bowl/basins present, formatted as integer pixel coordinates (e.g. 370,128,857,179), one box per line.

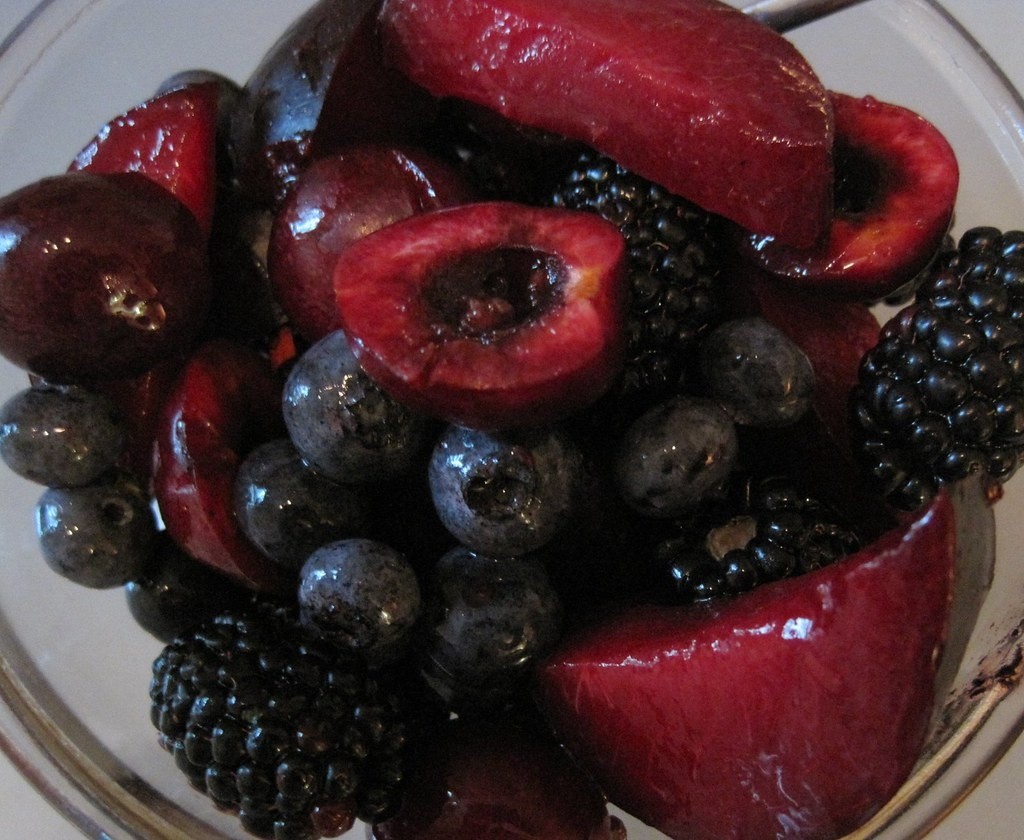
0,1,1024,839
